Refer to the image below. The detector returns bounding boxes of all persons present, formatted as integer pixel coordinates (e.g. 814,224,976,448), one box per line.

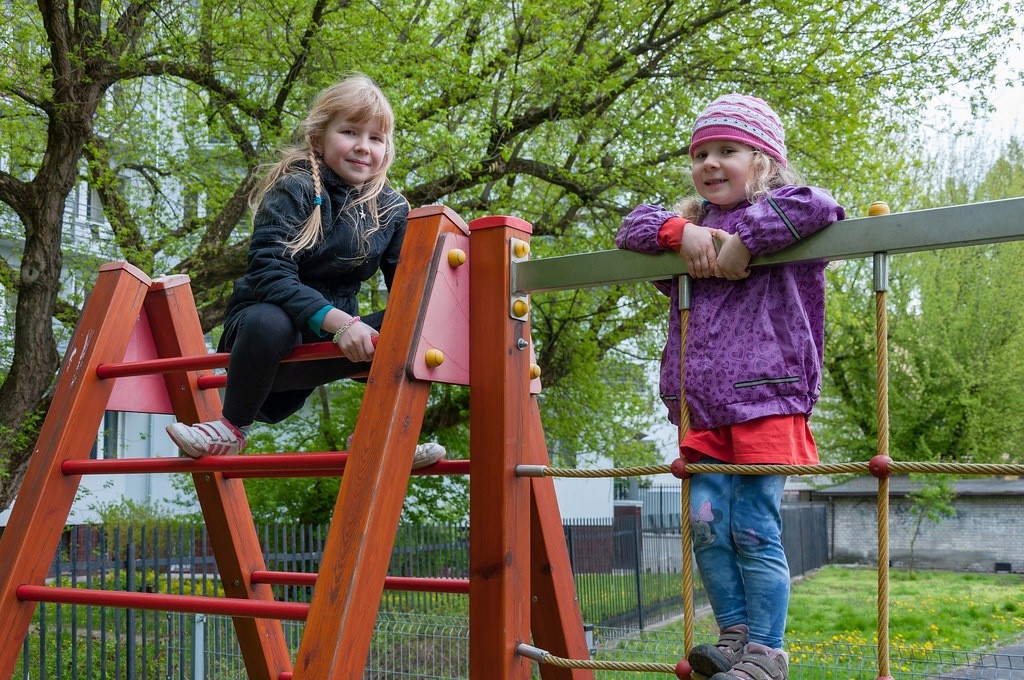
165,77,446,469
614,93,844,680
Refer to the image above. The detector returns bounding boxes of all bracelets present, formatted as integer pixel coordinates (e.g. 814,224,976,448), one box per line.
333,316,360,343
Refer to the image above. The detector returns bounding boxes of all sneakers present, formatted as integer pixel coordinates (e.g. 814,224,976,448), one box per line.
166,416,250,458
709,642,790,680
688,624,750,678
412,442,447,470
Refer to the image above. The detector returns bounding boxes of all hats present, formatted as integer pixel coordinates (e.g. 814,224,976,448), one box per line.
689,94,788,168
